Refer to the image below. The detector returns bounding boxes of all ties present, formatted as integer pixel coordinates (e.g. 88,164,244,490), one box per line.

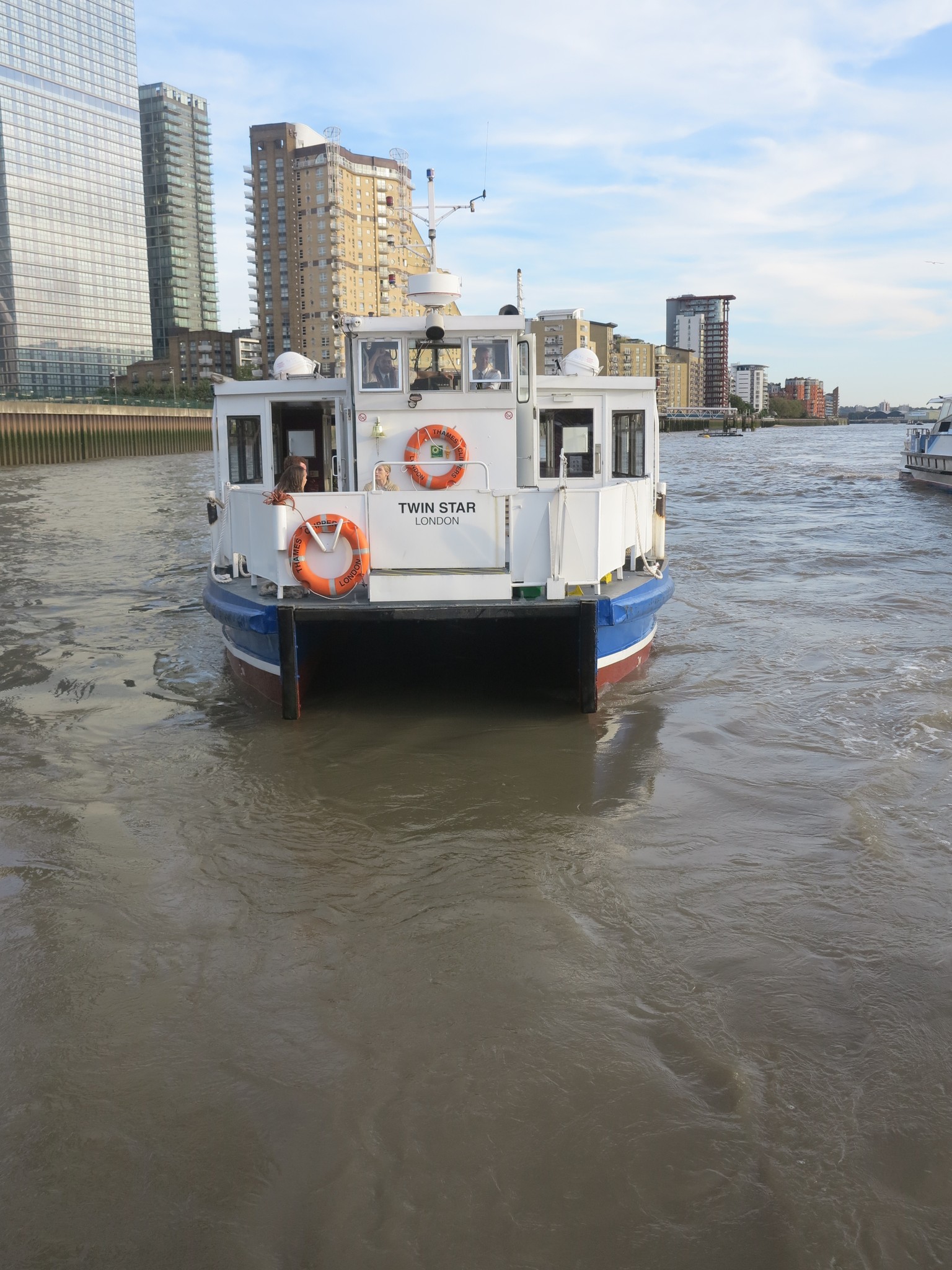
476,372,483,390
385,375,391,388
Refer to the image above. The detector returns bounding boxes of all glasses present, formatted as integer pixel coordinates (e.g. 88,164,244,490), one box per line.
378,359,392,365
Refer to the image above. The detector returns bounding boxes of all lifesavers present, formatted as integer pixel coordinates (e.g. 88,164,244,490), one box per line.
288,513,370,597
403,424,469,490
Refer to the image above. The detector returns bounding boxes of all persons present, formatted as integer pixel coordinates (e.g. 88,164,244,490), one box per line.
466,346,502,390
362,461,401,491
362,345,454,392
273,454,306,497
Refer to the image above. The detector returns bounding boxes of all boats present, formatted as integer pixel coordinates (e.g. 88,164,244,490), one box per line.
905,419,916,425
893,423,898,425
916,421,924,425
895,395,952,493
200,165,675,728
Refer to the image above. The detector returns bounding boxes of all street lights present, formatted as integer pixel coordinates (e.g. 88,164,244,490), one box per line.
168,366,176,405
110,371,118,405
